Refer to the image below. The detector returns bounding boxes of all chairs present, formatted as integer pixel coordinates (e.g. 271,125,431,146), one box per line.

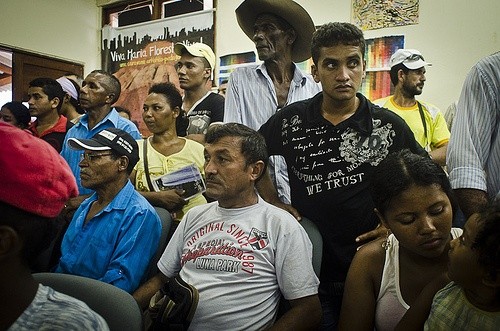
33,206,323,331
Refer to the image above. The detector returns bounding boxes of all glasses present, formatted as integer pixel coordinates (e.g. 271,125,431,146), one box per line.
80,152,122,165
402,54,425,63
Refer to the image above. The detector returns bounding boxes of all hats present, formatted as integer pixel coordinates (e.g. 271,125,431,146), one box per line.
68,128,140,172
235,0,316,63
389,49,433,69
174,43,217,71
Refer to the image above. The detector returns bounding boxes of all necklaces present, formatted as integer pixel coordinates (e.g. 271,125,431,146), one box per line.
64,205,67,207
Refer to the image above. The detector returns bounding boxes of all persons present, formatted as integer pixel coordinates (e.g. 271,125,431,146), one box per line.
0,0,500,331
173,41,225,145
127,82,207,224
27,77,74,154
424,201,500,331
251,21,430,331
132,122,323,331
58,70,143,211
366,48,450,167
111,107,131,120
224,0,322,205
332,149,464,331
56,127,163,296
0,121,111,331
56,77,86,128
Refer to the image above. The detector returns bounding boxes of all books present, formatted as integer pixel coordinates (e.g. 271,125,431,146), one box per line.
150,161,206,202
